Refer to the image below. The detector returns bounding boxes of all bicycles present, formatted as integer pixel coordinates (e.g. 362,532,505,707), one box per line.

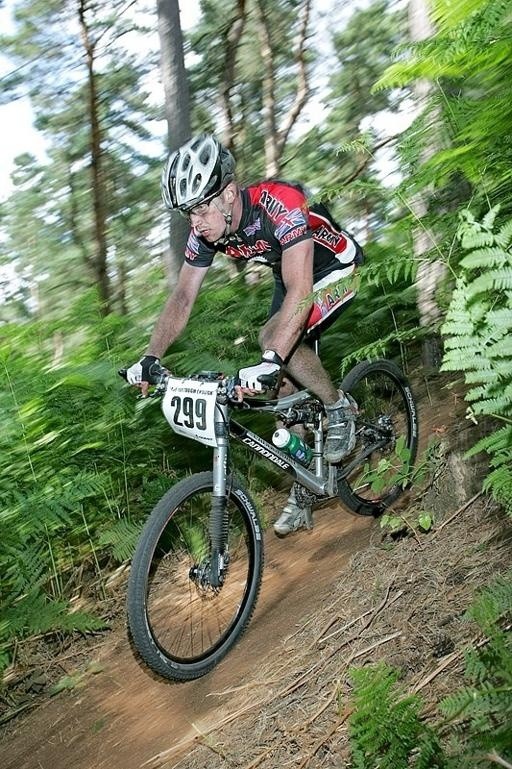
118,358,419,682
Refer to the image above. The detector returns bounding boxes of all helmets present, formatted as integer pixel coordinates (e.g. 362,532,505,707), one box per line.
160,133,235,210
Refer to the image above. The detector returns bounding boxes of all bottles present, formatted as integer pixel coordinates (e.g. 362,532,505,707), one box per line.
270,429,313,469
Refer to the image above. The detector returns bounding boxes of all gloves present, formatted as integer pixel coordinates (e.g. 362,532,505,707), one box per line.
126,353,160,385
236,350,283,392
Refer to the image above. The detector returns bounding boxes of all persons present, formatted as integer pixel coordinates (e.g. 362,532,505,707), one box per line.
127,136,366,534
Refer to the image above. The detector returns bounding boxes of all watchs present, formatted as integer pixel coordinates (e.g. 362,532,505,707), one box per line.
262,349,284,366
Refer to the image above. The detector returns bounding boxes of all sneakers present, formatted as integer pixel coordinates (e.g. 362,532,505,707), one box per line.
322,389,358,463
274,482,311,538
